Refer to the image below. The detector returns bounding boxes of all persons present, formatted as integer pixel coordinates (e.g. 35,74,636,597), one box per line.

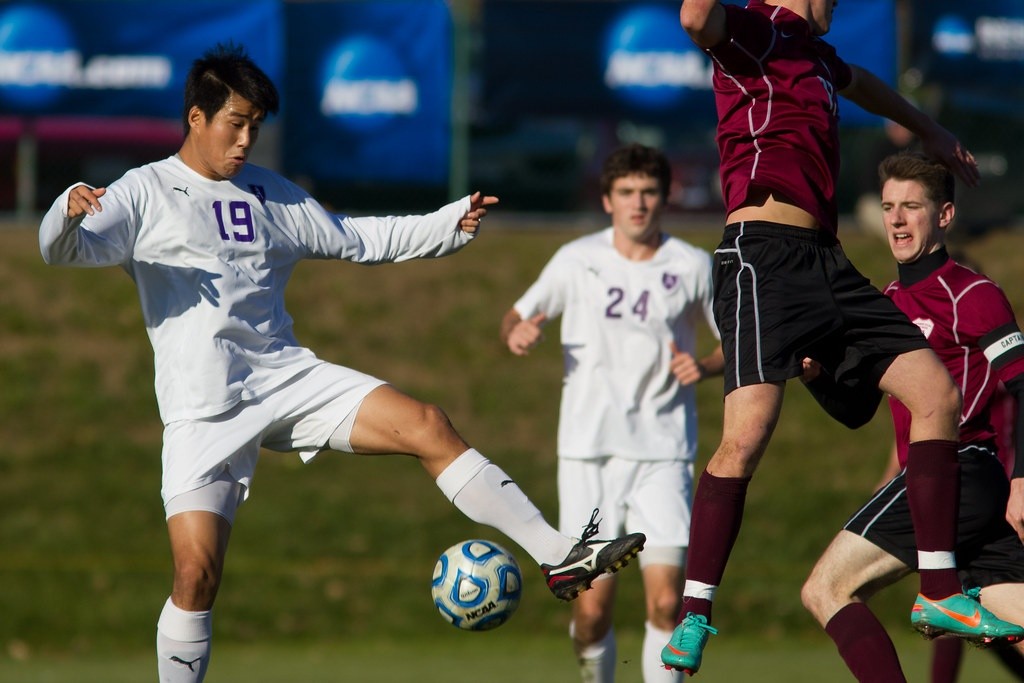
656,0,1024,677
36,36,646,683
501,142,726,683
798,156,1023,683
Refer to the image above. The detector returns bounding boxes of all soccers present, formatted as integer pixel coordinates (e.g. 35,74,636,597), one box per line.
431,538,523,633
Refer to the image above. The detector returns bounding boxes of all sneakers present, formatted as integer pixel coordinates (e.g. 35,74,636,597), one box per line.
911,585,1024,648
540,508,646,602
661,611,720,677
